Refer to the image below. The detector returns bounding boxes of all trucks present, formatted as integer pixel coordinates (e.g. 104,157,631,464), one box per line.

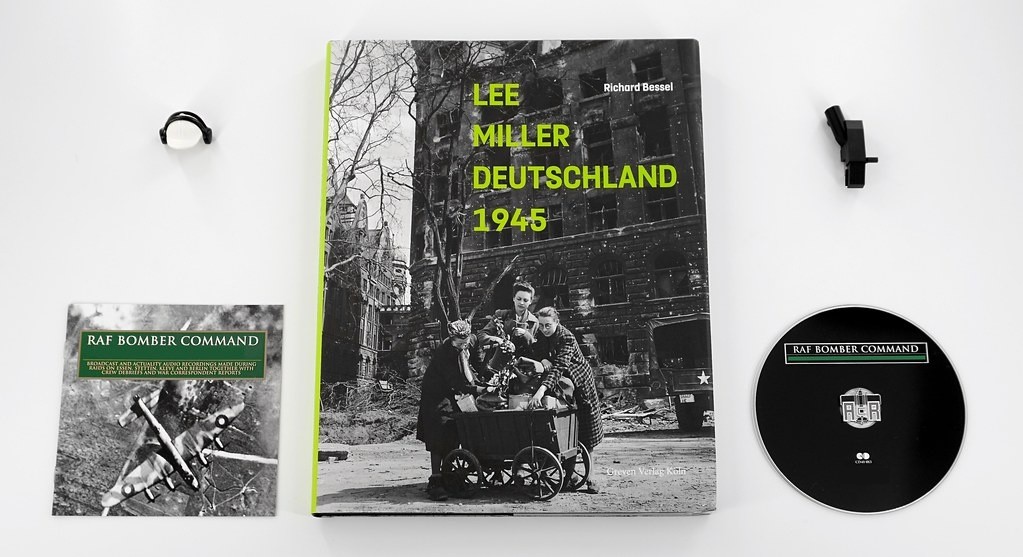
649,311,714,434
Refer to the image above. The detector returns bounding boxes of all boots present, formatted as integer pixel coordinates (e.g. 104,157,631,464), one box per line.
426,474,449,501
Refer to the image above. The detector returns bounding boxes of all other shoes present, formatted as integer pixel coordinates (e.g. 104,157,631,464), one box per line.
570,474,578,482
587,480,599,493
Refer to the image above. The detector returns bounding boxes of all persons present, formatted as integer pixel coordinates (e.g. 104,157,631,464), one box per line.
416,319,495,500
528,306,605,494
478,282,539,383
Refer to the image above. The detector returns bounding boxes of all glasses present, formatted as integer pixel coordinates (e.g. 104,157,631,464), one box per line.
538,323,551,330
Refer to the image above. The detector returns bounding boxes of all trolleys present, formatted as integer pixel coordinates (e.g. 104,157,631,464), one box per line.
426,366,593,502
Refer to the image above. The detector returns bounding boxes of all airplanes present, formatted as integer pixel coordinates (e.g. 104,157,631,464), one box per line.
100,388,247,507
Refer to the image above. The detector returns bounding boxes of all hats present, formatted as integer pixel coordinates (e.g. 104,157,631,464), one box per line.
447,318,472,339
